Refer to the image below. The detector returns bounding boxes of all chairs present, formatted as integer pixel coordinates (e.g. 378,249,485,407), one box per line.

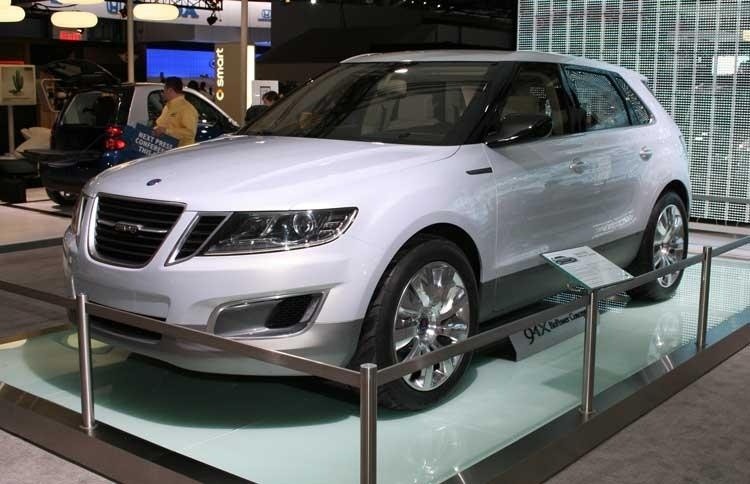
385,92,448,131
501,95,545,116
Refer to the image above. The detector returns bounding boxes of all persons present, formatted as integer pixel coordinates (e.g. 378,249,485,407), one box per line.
200,80,207,91
186,79,199,91
262,90,281,106
152,76,200,150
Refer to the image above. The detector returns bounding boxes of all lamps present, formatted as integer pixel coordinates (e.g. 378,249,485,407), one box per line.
207,9,218,25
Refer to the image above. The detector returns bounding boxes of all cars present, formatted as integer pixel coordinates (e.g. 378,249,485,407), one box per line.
42,81,239,208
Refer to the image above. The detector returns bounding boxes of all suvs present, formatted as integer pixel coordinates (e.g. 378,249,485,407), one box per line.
61,48,693,413
